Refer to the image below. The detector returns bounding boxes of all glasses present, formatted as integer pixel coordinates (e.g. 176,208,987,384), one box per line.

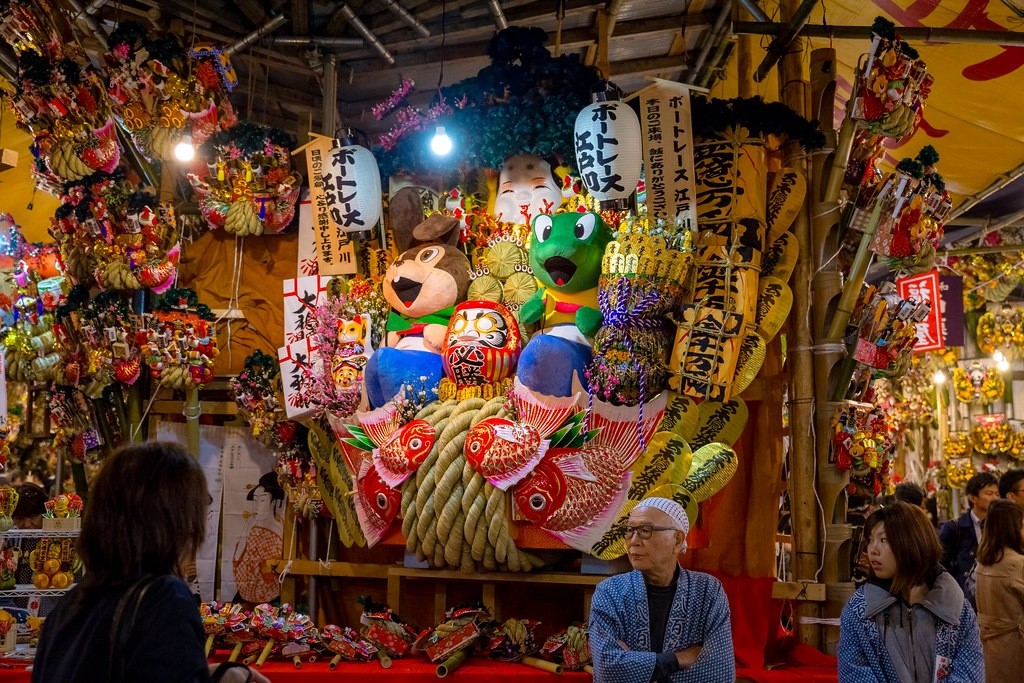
616,524,677,540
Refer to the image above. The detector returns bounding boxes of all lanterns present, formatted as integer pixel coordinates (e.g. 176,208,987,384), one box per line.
572,90,644,209
321,135,383,242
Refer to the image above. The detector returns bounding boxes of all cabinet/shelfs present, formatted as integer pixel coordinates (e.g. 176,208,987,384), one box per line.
266,465,628,624
0,528,83,598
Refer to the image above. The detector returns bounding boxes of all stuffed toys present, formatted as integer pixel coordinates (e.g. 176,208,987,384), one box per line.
324,150,672,554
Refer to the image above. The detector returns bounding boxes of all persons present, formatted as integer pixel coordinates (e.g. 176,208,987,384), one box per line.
836,503,986,683
30,441,274,683
4,466,77,620
588,496,736,683
938,469,1024,683
875,483,938,529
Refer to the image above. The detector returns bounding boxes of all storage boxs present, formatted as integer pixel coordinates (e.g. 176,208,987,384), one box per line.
277,275,337,421
43,517,80,530
